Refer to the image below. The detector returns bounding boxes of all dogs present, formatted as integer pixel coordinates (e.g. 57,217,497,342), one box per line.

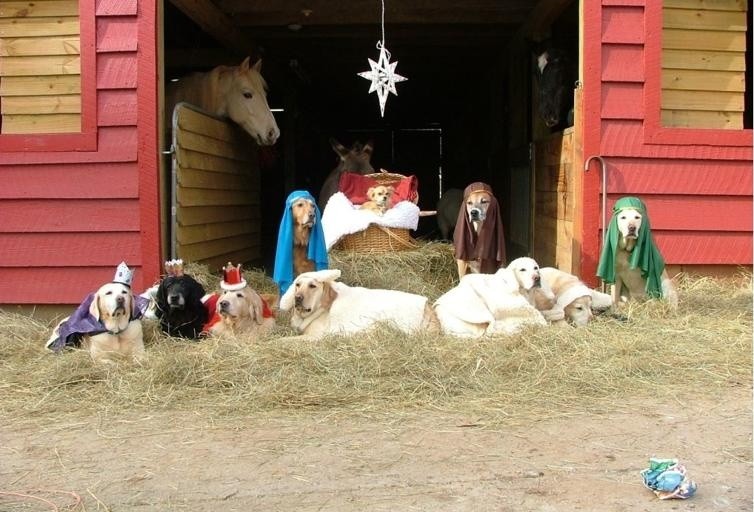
612,209,678,307
456,191,507,280
290,275,442,341
435,256,544,339
359,185,395,216
203,287,275,338
539,266,596,328
291,197,318,275
154,274,208,342
85,282,144,364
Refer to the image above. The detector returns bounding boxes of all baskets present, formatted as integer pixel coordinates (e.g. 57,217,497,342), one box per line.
330,169,420,252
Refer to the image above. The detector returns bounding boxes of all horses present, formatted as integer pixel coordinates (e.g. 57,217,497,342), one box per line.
165,56,281,148
